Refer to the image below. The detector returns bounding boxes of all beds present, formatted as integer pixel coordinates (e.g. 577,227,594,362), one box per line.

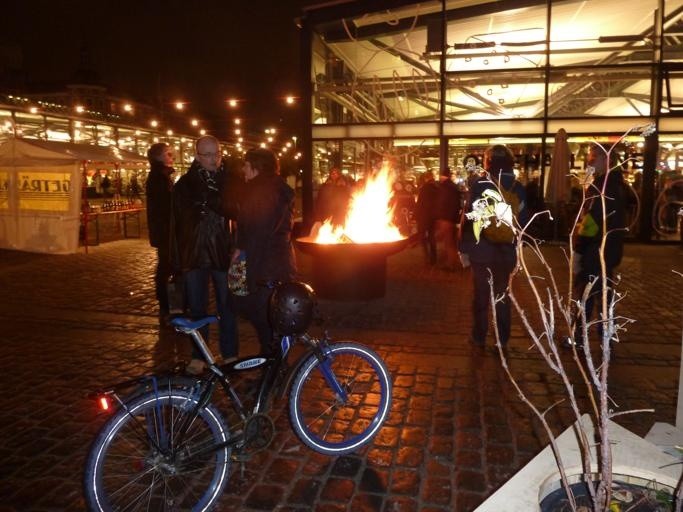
541,127,573,242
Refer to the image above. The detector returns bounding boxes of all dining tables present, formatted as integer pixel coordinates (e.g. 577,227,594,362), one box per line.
450,120,683,511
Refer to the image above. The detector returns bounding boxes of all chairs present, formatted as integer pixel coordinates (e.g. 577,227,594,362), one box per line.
560,335,581,349
187,359,206,374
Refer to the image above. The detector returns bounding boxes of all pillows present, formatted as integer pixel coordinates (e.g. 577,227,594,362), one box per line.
267,281,316,337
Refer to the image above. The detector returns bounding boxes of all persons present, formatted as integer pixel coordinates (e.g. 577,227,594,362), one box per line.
316,167,363,234
433,167,463,267
78,168,143,197
413,171,440,268
632,172,642,190
455,145,527,353
388,181,415,236
466,167,480,189
139,141,180,329
213,148,296,367
171,136,240,363
557,143,628,351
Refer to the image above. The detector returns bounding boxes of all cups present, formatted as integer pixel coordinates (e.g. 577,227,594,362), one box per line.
197,151,222,157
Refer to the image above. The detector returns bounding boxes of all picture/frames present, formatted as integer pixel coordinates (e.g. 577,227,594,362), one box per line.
82,208,147,245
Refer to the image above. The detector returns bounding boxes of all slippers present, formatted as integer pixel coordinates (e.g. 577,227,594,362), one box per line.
484,175,520,243
